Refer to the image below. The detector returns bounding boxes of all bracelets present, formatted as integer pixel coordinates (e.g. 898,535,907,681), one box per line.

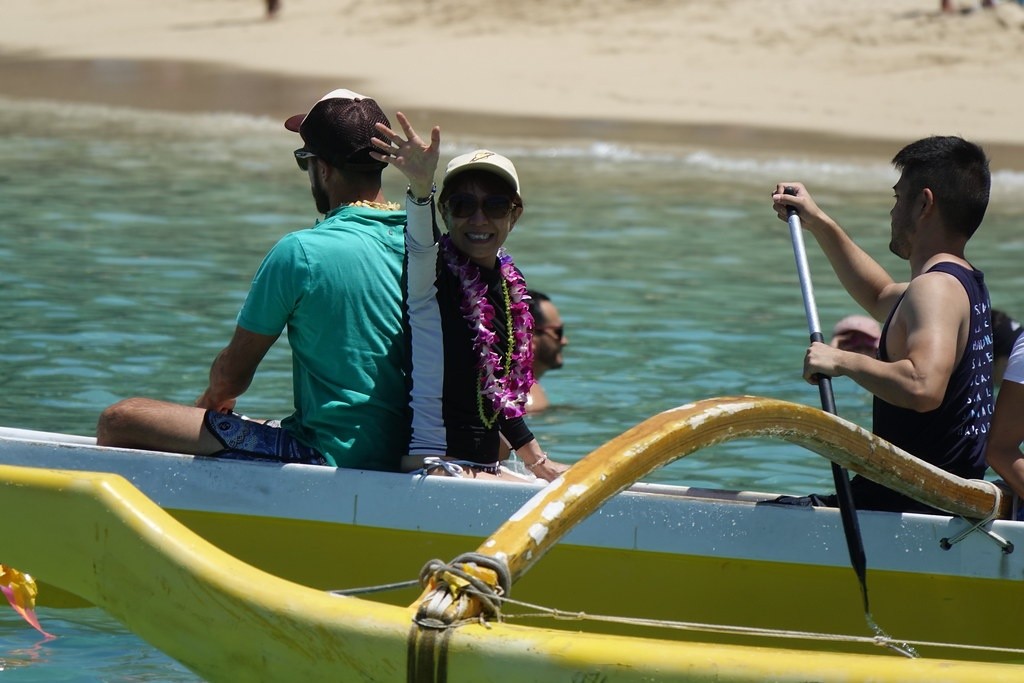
406,181,437,204
526,451,549,471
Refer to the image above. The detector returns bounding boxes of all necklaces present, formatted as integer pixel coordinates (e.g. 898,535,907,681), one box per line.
344,200,402,217
440,231,535,430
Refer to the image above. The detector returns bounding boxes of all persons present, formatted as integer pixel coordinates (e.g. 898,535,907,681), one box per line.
520,289,570,409
96,88,414,477
985,308,1024,384
984,332,1024,499
757,136,992,517
369,113,571,483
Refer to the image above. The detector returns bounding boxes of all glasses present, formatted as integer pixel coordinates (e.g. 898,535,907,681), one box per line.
294,148,319,171
536,324,563,342
445,190,515,218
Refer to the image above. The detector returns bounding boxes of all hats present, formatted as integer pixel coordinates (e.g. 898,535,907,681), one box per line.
434,150,522,214
833,316,880,349
284,89,392,171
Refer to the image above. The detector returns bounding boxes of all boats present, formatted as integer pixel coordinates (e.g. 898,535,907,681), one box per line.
0,394,1023,683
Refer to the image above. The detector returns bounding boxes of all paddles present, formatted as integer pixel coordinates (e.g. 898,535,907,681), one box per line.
782,186,871,617
